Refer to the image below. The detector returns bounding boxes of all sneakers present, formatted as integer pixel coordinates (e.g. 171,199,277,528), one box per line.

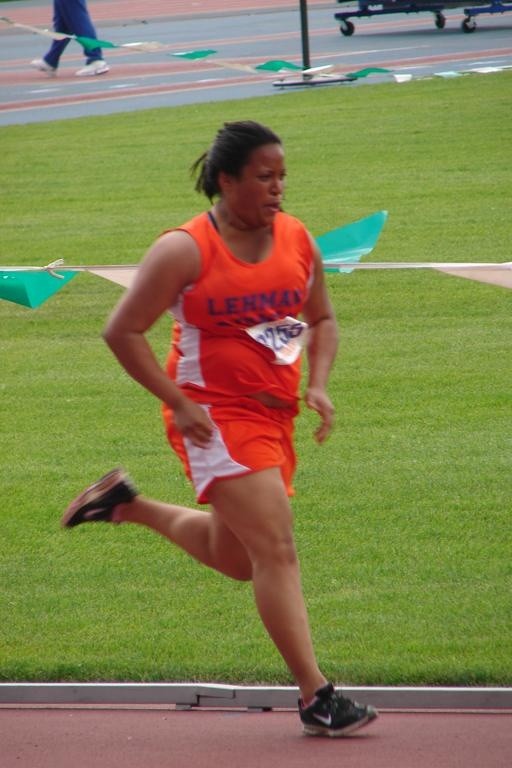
74,59,111,77
28,57,57,75
295,680,379,738
61,468,141,529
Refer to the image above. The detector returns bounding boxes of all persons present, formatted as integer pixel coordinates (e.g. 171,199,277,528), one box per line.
31,0,109,77
59,120,379,738
